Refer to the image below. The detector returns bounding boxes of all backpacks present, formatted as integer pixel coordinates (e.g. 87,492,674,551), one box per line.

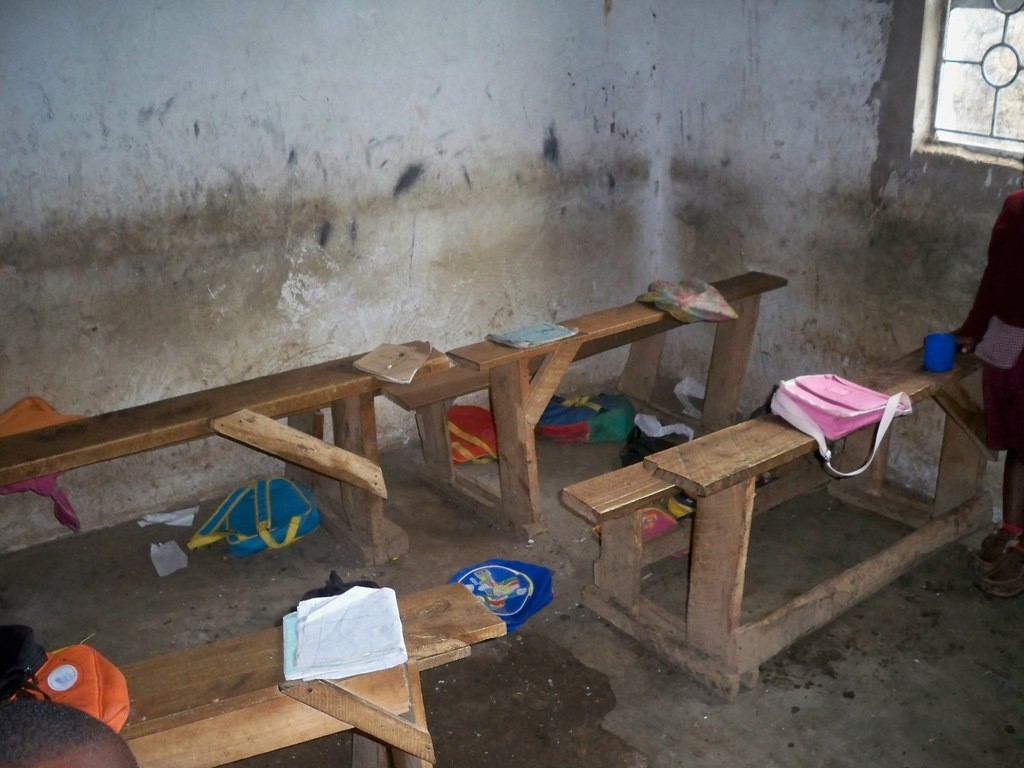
0,624,50,703
668,487,696,520
185,475,321,555
449,559,554,632
300,570,378,601
534,390,635,442
10,645,129,735
591,508,692,558
447,404,498,466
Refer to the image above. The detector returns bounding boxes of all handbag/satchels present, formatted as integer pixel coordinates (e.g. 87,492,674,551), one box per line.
974,315,1024,369
770,371,913,476
620,409,695,467
0,396,89,437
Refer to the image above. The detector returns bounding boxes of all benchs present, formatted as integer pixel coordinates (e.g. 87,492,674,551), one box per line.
561,461,835,618
384,328,668,511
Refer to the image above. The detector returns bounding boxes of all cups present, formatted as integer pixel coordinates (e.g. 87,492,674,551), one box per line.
922,333,955,373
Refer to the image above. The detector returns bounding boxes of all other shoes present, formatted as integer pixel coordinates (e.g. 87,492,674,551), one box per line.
978,533,1024,595
968,521,1022,577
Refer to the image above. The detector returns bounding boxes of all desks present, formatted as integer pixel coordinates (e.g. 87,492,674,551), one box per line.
640,341,995,705
444,268,790,537
119,585,507,768
1,345,452,568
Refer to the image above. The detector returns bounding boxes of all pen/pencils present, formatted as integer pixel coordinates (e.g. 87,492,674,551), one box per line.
386,354,409,369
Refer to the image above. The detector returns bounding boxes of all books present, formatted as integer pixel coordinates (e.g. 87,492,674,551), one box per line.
282,586,407,682
353,341,433,384
485,322,580,349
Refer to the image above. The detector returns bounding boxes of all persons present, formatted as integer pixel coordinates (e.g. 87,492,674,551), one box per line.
924,155,1024,598
0,700,138,768
